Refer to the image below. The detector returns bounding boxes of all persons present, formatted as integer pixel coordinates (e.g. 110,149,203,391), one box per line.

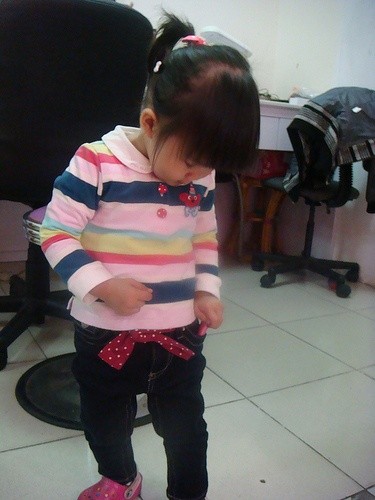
40,8,260,500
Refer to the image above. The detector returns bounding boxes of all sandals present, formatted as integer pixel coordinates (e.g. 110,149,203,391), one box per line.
77,472,142,500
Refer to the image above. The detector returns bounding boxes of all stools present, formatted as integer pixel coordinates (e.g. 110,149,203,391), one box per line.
15,204,156,432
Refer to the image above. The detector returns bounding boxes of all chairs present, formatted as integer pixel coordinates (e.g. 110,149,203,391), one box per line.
251,86,375,299
0,0,157,370
